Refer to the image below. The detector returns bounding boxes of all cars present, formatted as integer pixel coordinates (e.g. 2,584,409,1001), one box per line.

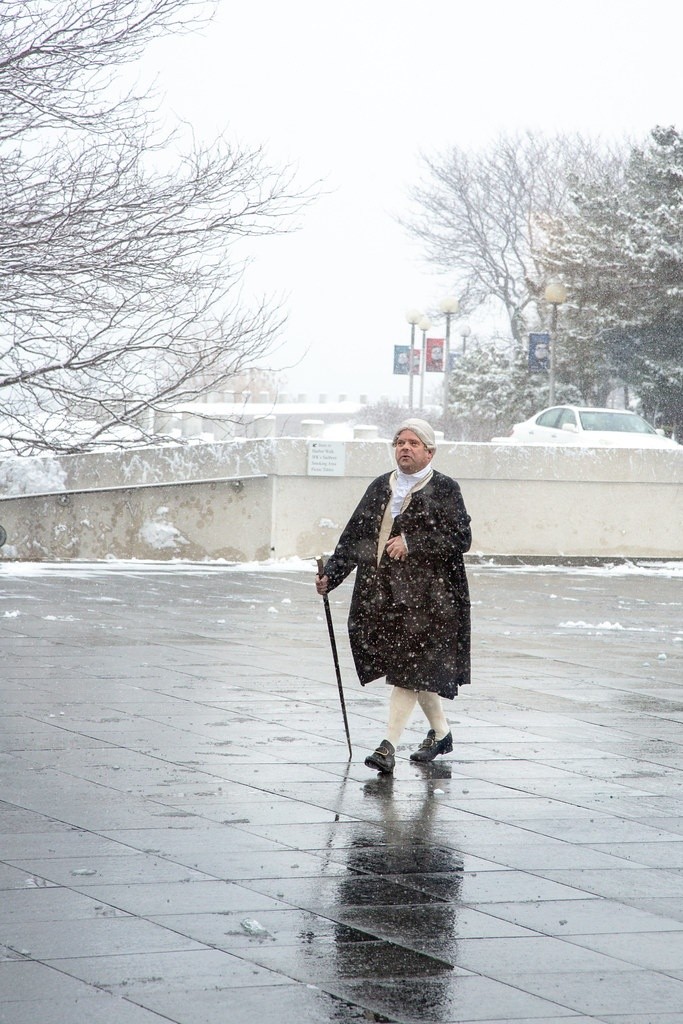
511,405,682,449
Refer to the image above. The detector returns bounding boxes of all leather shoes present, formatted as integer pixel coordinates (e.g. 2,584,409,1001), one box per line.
410,728,453,762
365,739,395,774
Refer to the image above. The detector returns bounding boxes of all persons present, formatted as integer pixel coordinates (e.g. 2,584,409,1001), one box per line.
314,418,473,775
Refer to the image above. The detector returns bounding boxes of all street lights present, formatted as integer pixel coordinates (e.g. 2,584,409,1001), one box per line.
545,282,567,409
440,295,460,412
417,315,432,410
405,308,423,408
458,324,471,355
